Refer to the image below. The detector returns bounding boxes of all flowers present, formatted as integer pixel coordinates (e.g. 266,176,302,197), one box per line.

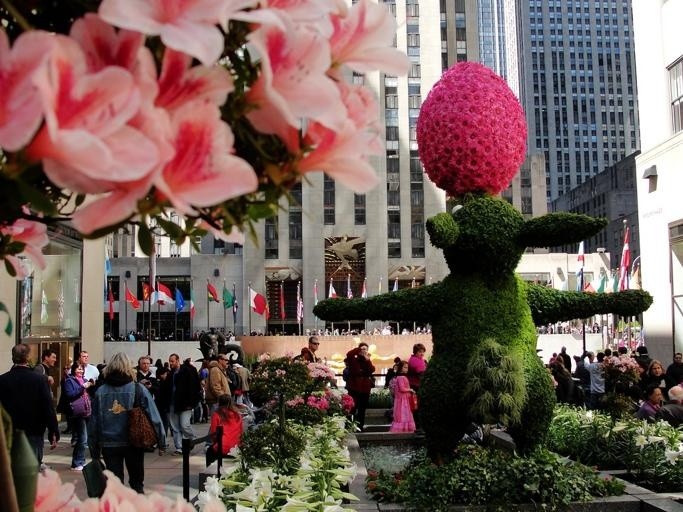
253,350,311,431
301,361,337,396
267,389,357,426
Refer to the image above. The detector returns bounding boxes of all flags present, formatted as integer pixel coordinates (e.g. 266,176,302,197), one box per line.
575,227,641,292
107,279,416,320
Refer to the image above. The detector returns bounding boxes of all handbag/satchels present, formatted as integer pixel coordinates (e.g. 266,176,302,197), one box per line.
128,382,158,452
83,459,106,497
409,393,418,411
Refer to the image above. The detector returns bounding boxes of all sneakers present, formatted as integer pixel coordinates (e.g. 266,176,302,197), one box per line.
40,462,53,472
172,451,182,456
71,465,84,471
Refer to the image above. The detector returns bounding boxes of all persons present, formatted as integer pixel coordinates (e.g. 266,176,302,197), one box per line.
0,328,429,498
536,321,683,427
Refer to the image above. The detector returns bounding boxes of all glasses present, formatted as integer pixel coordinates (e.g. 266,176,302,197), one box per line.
312,342,319,345
